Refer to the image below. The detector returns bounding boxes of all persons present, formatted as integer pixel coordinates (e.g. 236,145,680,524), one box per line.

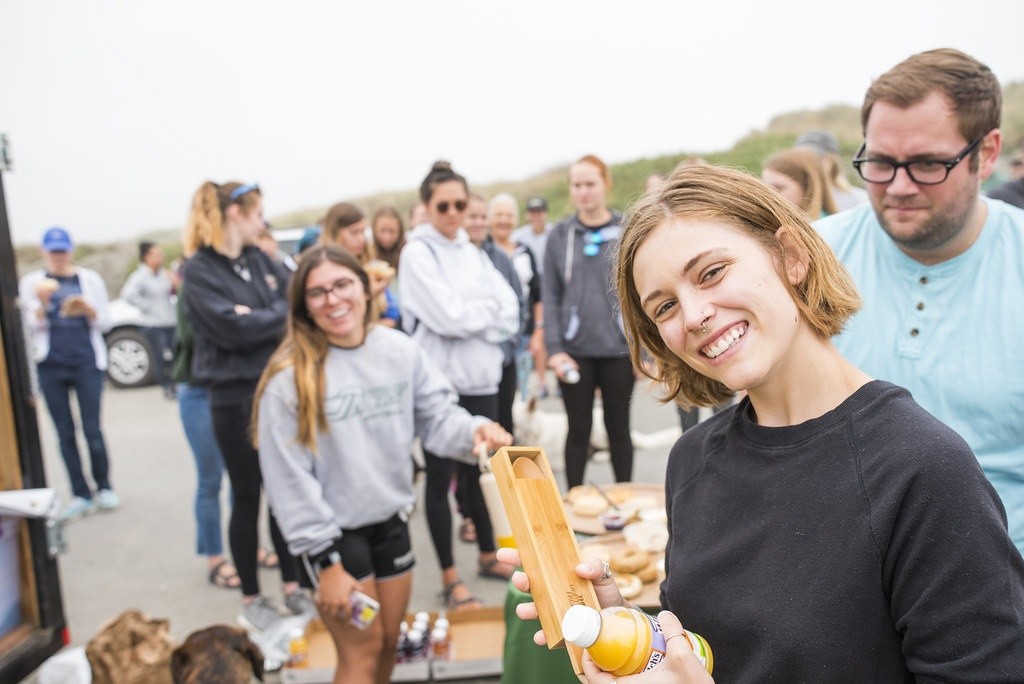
295,192,559,411
760,125,871,227
400,161,521,615
987,150,1024,211
118,239,185,391
254,244,514,684
495,166,1024,684
543,154,735,485
807,49,1024,553
180,183,316,629
19,229,120,522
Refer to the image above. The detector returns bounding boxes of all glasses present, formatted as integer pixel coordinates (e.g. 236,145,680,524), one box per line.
305,276,358,309
434,198,470,214
852,125,999,185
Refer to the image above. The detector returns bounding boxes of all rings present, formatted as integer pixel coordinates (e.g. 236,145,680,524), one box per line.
665,632,686,642
596,561,612,582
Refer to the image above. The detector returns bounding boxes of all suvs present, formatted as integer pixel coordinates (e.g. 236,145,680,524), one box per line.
100,228,320,388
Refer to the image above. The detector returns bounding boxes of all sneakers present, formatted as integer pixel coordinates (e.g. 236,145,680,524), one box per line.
56,487,120,524
281,586,318,614
237,595,285,632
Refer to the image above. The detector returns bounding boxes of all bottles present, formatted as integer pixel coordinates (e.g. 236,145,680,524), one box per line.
561,606,713,681
553,358,580,385
393,612,452,666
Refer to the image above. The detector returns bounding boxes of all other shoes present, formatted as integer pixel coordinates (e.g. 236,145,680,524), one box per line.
535,383,563,399
459,523,477,542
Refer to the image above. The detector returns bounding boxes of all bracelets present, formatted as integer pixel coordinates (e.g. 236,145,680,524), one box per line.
313,551,340,569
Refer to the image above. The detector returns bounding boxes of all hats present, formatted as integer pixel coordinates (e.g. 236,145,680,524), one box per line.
796,130,840,155
525,197,547,211
42,227,74,251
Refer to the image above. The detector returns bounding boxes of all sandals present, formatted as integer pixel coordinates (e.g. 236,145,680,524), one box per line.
478,557,509,581
256,546,280,567
208,560,242,588
443,580,486,611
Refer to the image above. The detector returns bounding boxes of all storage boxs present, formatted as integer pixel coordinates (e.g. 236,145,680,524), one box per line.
284,614,431,682
432,604,509,679
488,448,604,677
